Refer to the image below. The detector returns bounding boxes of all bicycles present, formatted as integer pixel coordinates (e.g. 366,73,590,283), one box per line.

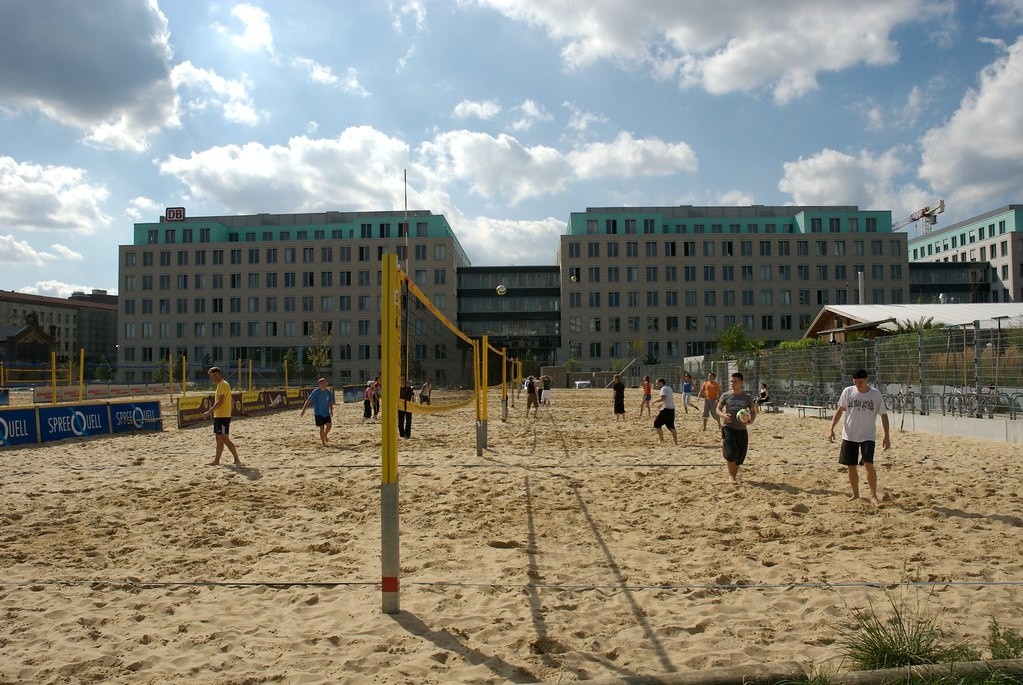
768,381,998,413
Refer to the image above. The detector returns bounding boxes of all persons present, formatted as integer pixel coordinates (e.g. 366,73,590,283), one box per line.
612,374,625,422
754,382,769,414
202,366,241,466
679,366,699,414
697,372,722,432
828,369,890,508
525,376,539,418
652,378,678,446
638,376,651,416
715,372,756,485
535,375,553,407
363,377,434,421
398,377,415,438
301,378,334,446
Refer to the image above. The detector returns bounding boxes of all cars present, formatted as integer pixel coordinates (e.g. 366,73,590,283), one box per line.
521,380,540,389
574,380,592,390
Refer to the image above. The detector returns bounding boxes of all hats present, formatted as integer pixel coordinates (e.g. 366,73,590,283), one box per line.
367,380,374,385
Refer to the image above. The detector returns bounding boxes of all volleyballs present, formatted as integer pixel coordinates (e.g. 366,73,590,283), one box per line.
496,284,506,295
570,276,577,283
737,408,752,423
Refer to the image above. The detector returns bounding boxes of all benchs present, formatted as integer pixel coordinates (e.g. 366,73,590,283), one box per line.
794,404,831,421
759,402,776,414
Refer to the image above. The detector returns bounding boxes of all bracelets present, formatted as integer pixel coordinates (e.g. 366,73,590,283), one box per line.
209,409,213,414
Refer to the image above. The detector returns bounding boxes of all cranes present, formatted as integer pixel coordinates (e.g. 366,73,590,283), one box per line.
892,199,946,234
